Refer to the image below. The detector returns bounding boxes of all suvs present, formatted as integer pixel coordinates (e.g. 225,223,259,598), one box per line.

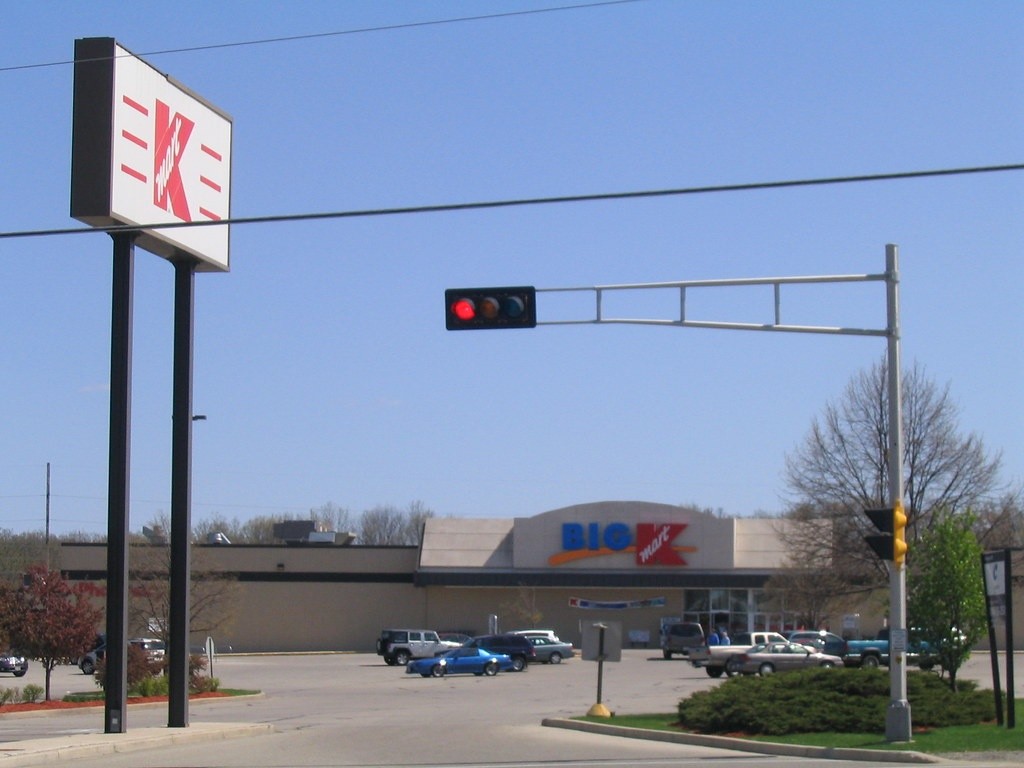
377,627,466,667
460,634,537,672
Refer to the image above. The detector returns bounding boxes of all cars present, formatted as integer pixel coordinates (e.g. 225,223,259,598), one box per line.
76,638,166,676
528,635,577,664
732,640,846,678
438,632,470,643
779,630,848,659
509,630,560,643
405,644,514,678
911,623,968,652
1,653,29,678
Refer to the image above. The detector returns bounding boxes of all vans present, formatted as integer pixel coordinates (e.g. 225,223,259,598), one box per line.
659,621,706,660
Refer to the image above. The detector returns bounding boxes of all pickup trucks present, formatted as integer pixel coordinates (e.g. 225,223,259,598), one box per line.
828,625,947,671
693,630,793,679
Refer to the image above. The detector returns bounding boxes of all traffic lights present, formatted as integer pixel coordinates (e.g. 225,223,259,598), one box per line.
442,283,538,330
859,498,911,568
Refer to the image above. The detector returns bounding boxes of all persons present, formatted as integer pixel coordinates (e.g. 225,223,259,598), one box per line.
720,632,730,645
709,629,720,645
